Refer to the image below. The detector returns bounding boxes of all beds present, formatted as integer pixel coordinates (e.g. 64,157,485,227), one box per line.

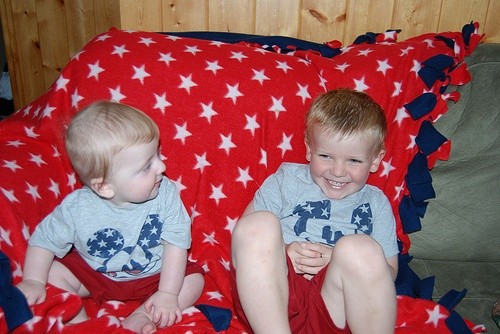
0,26,500,334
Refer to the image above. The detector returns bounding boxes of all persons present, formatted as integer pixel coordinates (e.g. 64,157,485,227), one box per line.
229,87,401,334
13,101,205,334
